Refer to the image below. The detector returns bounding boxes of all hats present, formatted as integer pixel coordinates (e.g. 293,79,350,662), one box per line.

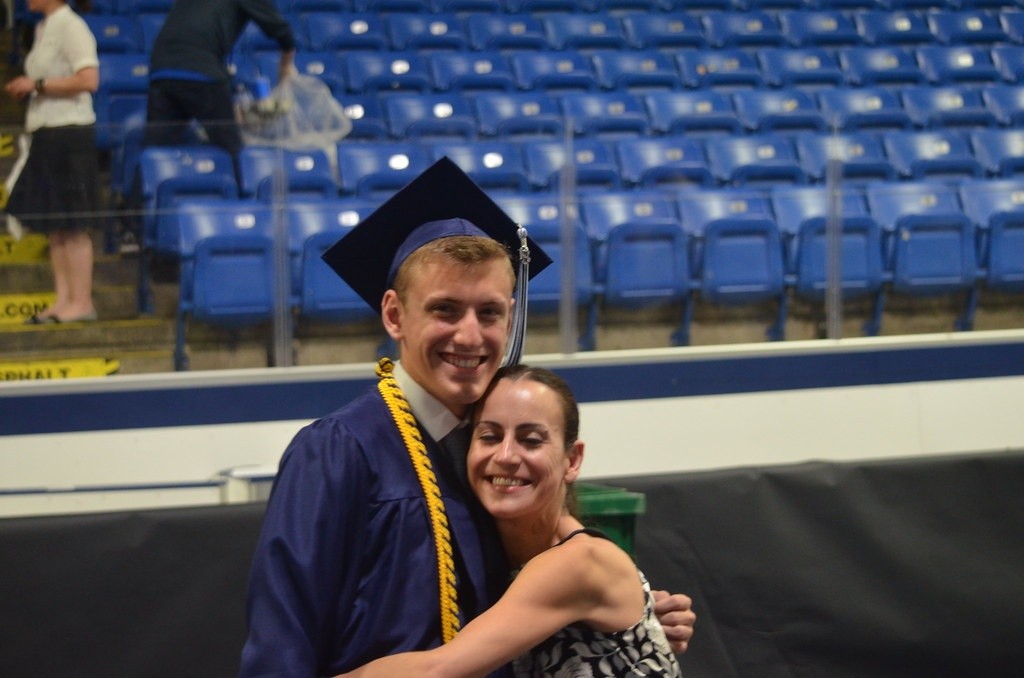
320,155,553,368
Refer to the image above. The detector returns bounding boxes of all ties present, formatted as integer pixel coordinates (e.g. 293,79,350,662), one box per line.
442,428,512,607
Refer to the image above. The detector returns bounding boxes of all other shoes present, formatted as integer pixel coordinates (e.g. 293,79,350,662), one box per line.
121,233,140,254
24,308,98,325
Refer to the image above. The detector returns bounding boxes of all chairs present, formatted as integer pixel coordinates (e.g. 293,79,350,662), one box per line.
20,0,1024,371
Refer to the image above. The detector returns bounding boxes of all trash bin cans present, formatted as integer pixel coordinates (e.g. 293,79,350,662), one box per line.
566,482,646,559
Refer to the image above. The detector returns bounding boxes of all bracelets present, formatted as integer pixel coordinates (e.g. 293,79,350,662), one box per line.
35,78,46,93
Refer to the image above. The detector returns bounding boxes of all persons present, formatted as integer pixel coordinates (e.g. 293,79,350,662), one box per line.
4,0,108,324
141,0,295,284
239,157,696,678
337,364,682,678
